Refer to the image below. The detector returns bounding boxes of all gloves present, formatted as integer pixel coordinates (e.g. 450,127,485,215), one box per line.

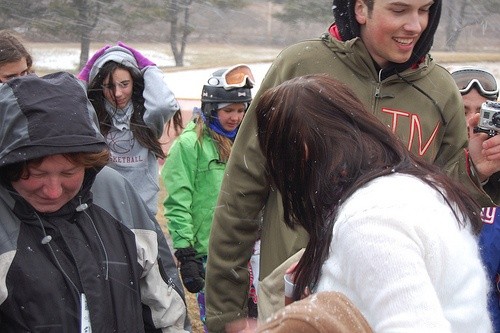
117,41,156,71
174,246,205,293
77,45,111,83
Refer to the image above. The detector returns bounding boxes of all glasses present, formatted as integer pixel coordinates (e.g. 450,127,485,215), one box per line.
221,65,255,91
448,68,499,100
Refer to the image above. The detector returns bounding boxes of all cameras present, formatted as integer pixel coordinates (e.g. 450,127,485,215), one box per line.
477,100,500,132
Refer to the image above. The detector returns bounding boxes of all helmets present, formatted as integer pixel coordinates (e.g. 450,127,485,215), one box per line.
201,66,253,103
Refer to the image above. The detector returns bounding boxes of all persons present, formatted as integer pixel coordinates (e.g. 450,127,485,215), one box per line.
448,66,499,333
159,62,259,319
256,72,494,333
0,30,35,83
204,0,499,332
74,41,183,216
0,71,199,333
